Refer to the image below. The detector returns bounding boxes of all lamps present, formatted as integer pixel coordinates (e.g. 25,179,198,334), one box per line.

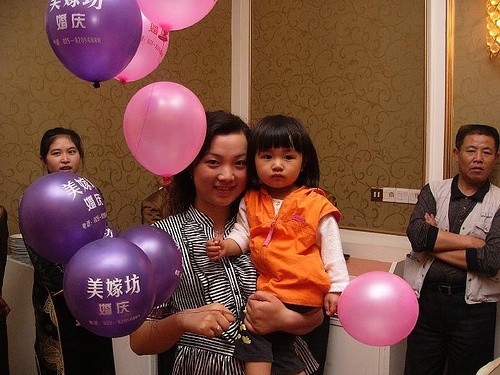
486,0,500,60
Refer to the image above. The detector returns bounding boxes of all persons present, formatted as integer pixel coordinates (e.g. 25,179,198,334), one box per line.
207,115,349,375
405,125,500,375
0,205,10,375
130,111,324,375
18,127,116,375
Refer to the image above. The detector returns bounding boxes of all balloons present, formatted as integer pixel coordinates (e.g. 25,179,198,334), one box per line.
123,83,206,185
337,271,419,347
18,172,182,337
45,0,218,87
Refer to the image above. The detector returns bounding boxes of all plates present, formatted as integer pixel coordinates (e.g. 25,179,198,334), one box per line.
9,234,31,262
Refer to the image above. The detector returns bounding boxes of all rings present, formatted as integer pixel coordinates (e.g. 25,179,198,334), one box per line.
214,325,220,331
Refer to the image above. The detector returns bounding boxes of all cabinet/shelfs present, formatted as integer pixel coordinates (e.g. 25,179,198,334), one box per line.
0,242,408,375
